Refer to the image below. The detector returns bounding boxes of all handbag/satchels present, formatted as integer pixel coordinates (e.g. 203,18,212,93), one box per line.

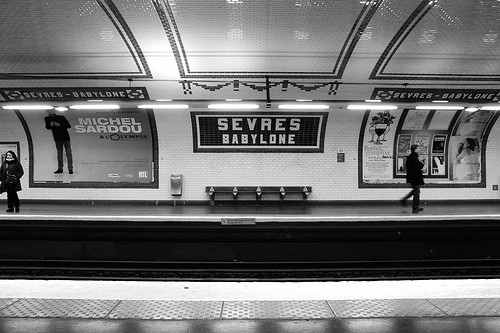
3,174,19,190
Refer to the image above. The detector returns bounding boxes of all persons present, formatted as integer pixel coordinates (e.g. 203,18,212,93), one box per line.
451,138,480,182
0,150,24,213
400,145,426,212
44,109,74,174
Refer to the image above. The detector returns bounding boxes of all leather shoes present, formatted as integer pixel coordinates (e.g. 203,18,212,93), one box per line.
5,207,14,212
400,198,408,207
411,206,423,212
68,169,73,174
16,207,19,212
53,168,63,173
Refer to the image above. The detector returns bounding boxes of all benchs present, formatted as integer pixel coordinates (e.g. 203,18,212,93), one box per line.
206,186,312,200
